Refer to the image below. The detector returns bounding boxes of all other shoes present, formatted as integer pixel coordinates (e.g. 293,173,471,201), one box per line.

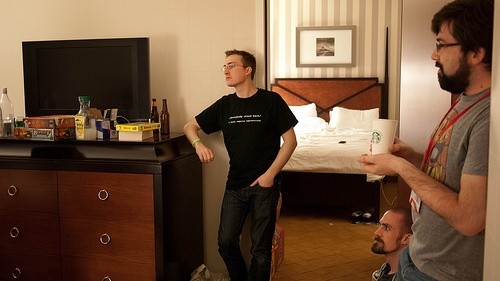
351,204,377,224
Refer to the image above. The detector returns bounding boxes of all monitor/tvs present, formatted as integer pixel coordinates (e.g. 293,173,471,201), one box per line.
22,38,151,121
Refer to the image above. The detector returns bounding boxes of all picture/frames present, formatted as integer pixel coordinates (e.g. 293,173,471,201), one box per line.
295,25,357,67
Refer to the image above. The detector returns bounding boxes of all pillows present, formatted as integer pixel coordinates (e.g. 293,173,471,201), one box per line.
289,102,318,119
328,106,380,132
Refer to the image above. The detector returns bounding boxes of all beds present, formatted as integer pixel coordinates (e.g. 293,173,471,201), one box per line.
270,77,387,223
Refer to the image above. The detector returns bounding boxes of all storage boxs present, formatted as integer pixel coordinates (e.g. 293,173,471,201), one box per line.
118,130,154,142
270,224,284,280
23,115,76,128
14,127,75,141
116,122,161,132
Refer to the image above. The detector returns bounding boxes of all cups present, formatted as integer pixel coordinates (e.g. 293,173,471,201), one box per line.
370,118,399,155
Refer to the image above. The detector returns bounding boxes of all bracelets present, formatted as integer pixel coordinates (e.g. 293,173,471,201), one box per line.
192,138,200,148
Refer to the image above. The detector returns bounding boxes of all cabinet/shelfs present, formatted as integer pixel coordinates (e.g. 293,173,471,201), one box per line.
0,138,203,281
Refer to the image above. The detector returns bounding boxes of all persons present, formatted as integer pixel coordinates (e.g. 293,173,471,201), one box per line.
371,204,413,281
182,48,298,281
355,0,490,281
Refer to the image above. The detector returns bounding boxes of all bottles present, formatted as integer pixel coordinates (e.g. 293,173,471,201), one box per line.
151,98,159,134
0,88,14,138
161,99,169,135
74,96,97,141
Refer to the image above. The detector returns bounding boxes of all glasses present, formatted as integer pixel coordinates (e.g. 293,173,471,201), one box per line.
220,64,245,72
435,40,461,53
